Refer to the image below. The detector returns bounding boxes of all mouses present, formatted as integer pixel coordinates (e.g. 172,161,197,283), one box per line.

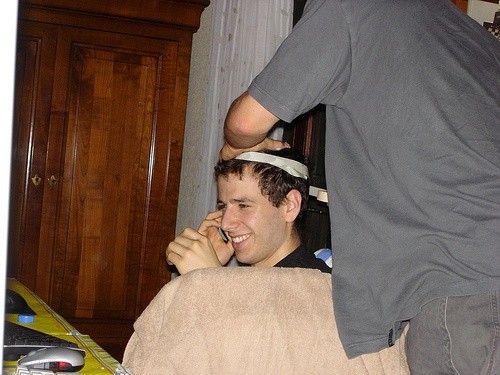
17,346,85,373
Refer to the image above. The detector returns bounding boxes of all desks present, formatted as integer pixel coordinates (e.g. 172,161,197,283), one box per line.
3,278,125,374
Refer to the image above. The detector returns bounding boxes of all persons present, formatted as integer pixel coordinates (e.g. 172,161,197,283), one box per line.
164,0,500,375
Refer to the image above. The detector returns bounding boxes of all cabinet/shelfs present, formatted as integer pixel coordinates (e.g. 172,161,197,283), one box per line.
9,1,197,321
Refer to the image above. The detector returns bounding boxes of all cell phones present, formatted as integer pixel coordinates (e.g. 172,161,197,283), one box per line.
219,228,228,243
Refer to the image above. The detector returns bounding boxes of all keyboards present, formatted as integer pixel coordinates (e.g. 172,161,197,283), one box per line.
17,366,77,375
3,320,86,361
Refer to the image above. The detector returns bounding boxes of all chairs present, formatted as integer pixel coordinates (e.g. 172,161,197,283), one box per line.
122,267,410,375
